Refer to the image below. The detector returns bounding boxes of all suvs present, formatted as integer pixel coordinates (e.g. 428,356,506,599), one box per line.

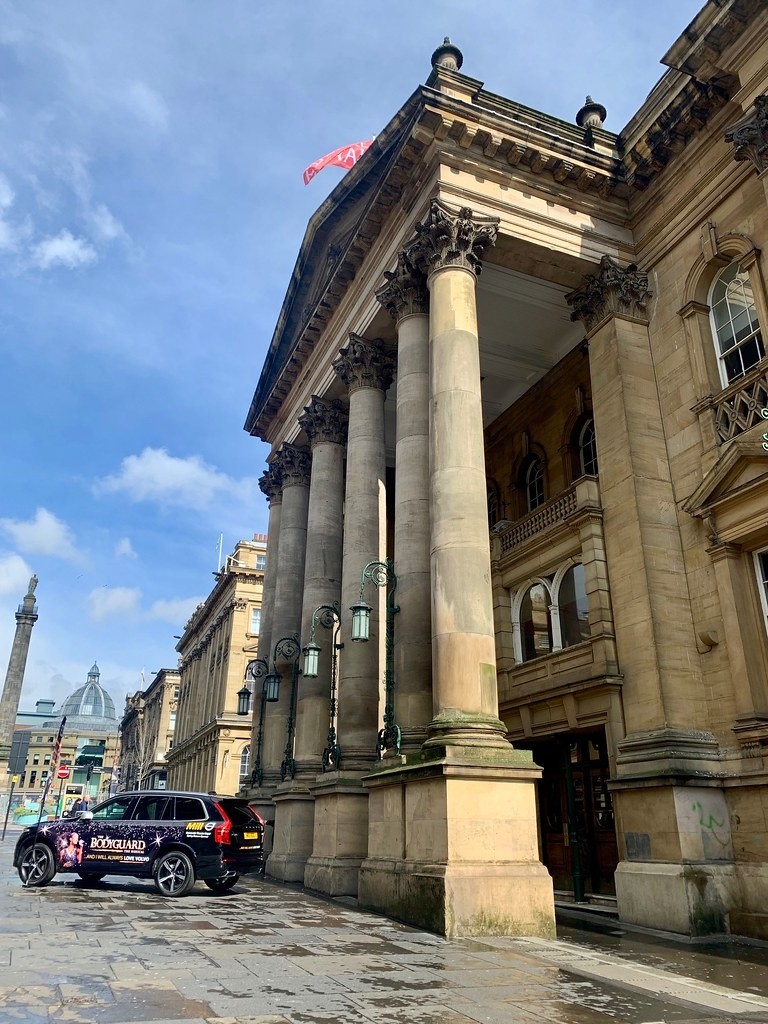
12,790,266,898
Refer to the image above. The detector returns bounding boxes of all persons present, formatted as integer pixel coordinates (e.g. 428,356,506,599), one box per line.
71,796,89,816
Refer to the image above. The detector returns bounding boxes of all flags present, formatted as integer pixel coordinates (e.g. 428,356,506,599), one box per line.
302,139,372,185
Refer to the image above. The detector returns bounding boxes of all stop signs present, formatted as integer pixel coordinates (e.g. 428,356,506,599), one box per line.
55,764,71,780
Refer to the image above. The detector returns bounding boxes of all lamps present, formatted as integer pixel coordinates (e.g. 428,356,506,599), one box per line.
302,600,346,772
350,561,401,758
265,633,301,783
236,653,268,787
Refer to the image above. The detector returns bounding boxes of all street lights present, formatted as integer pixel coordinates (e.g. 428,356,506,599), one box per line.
350,558,400,762
262,632,301,783
237,658,269,789
302,599,343,774
